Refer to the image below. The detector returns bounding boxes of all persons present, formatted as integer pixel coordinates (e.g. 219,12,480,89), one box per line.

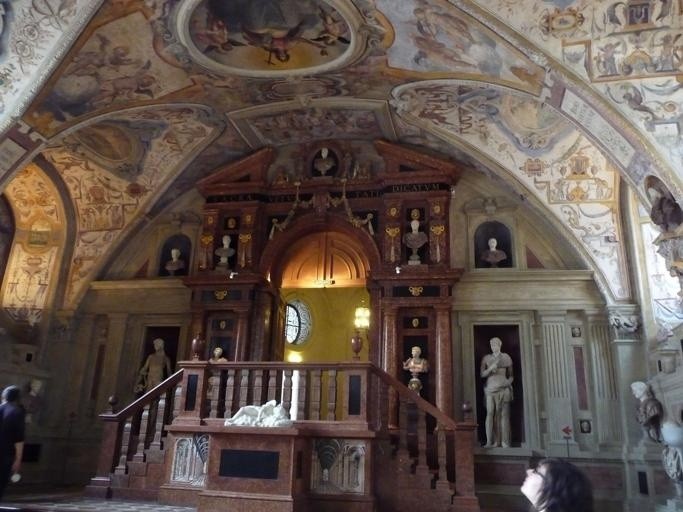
65,44,157,107
164,248,184,270
2,385,26,493
318,5,336,46
223,398,295,428
215,236,234,256
413,3,481,70
314,147,334,170
132,338,173,396
269,36,290,63
483,238,508,263
196,15,235,52
480,337,515,448
630,382,662,425
519,457,593,512
208,347,228,363
403,219,428,247
401,347,430,373
